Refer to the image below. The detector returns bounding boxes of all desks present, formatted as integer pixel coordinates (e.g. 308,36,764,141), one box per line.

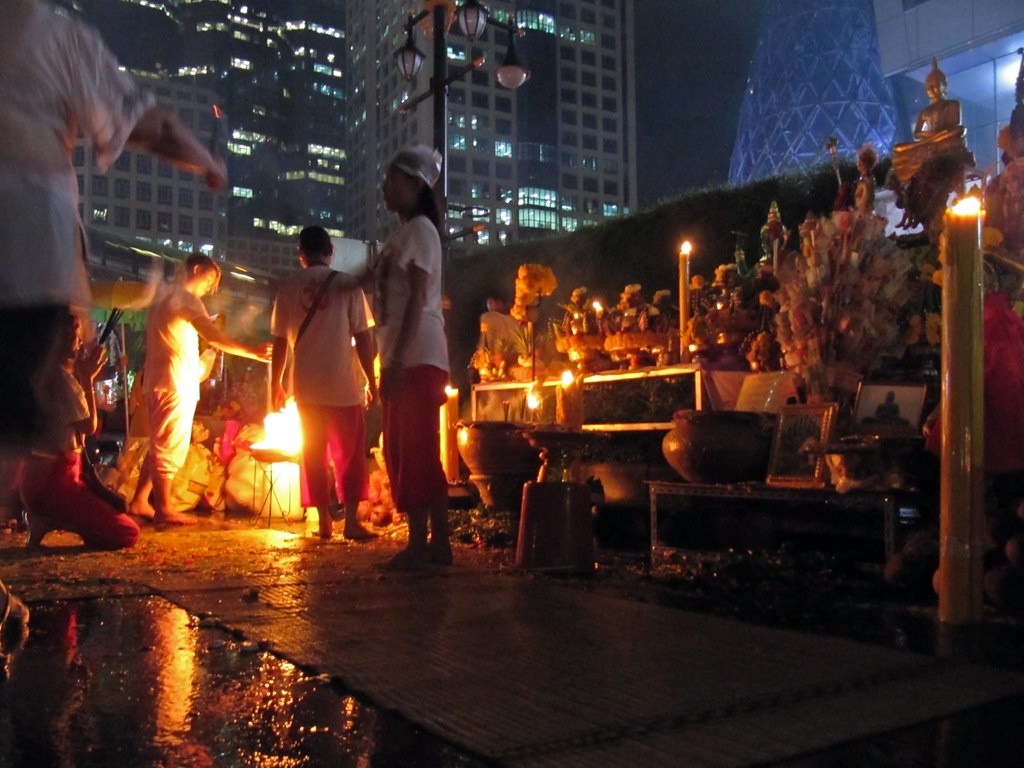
643,480,899,571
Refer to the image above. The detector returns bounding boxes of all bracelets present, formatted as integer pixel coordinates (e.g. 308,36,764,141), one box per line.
390,357,407,370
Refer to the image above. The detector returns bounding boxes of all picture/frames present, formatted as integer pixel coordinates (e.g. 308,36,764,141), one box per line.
849,380,928,434
765,402,837,488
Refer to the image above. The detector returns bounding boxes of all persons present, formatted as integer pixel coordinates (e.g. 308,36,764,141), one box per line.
838,148,876,213
128,250,274,526
892,57,965,153
269,225,378,540
733,202,801,286
1,0,229,552
465,290,526,385
343,143,454,573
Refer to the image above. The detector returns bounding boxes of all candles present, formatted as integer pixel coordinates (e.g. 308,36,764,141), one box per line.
941,195,985,626
439,384,460,482
678,239,694,354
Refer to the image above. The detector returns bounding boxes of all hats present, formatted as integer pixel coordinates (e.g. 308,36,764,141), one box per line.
392,144,443,190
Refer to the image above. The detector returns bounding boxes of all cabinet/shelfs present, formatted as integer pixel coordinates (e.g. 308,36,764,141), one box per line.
466,366,708,433
